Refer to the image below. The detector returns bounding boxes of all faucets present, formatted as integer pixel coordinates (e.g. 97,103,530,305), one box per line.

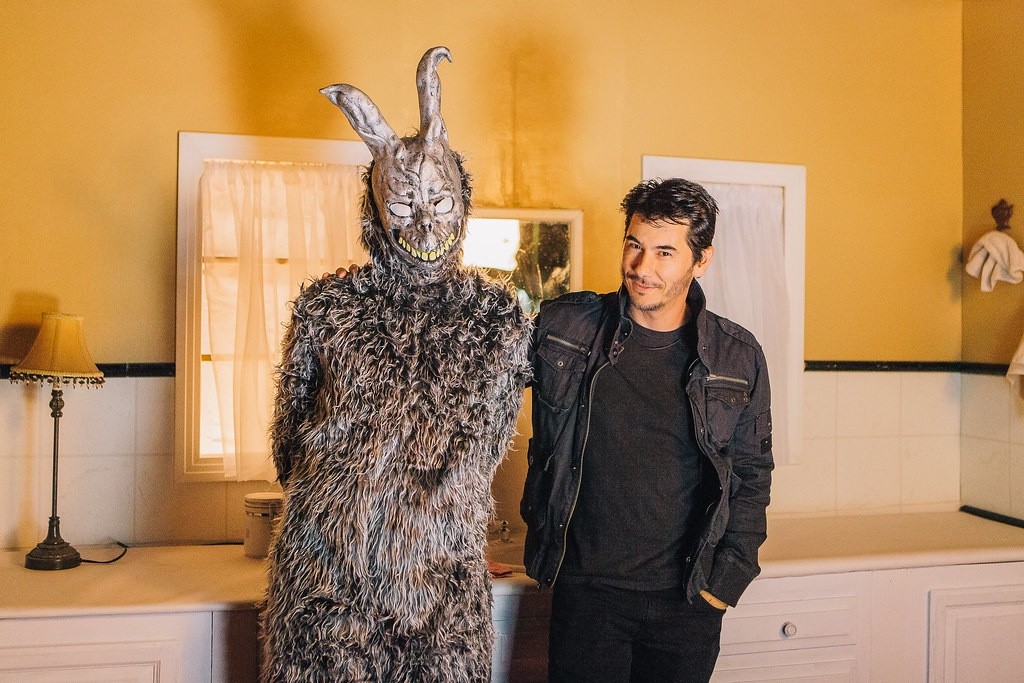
488,519,514,544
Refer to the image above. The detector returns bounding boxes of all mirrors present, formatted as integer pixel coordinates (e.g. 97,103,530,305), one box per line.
462,208,583,314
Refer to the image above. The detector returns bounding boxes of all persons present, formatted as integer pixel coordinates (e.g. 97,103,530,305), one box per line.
261,46,531,683
322,179,776,683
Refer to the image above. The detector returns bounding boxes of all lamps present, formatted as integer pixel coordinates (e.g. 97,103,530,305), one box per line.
9,312,106,570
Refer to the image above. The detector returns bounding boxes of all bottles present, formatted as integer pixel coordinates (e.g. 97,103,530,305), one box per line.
243,492,284,559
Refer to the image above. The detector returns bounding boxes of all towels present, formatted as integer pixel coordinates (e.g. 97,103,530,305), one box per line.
965,229,1024,293
1005,334,1024,403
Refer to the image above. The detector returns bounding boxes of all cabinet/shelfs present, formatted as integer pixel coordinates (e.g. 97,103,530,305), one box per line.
0,561,1024,683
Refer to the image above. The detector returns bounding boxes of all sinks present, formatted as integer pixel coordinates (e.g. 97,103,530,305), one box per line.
487,546,524,566
